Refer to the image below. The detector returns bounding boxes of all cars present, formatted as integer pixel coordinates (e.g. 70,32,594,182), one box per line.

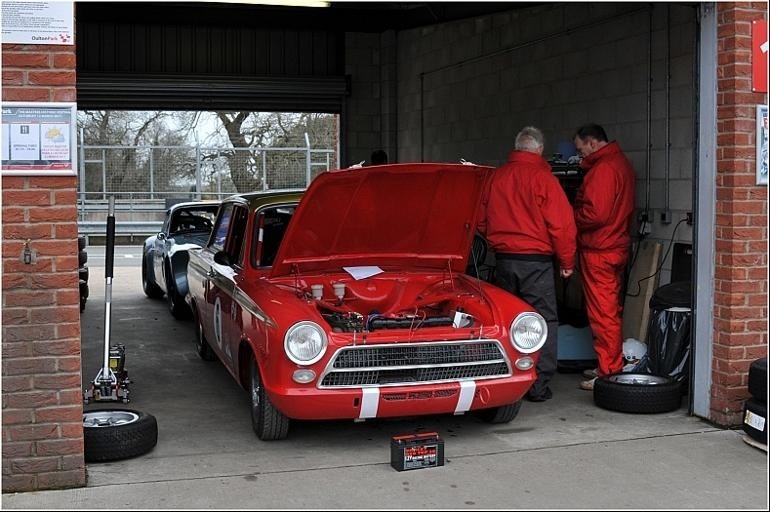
186,161,549,440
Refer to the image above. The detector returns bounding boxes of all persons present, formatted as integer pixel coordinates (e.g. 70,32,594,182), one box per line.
371,147,387,165
476,125,579,401
571,123,636,391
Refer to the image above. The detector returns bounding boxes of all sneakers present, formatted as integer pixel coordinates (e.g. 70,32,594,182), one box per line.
583,369,601,379
528,386,553,403
580,375,599,391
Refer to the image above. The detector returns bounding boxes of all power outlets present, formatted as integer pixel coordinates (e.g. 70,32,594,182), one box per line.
639,222,651,235
687,212,693,224
639,209,654,222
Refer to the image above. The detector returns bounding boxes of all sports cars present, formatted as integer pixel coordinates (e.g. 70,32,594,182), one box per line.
141,201,222,316
